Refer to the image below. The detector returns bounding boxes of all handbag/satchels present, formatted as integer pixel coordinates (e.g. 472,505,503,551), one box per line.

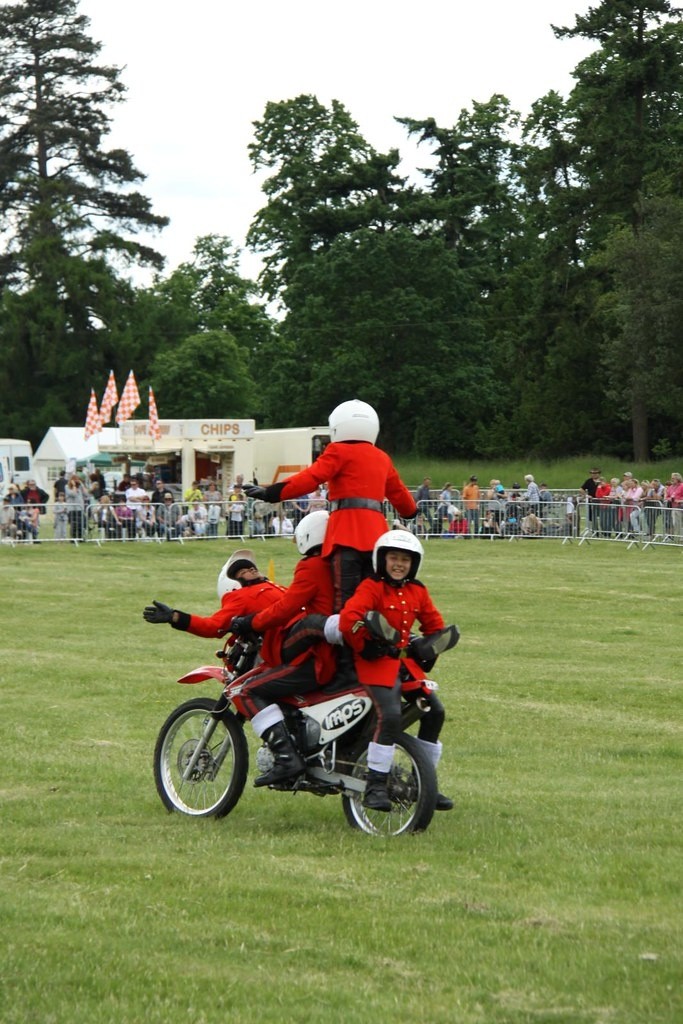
447,504,461,516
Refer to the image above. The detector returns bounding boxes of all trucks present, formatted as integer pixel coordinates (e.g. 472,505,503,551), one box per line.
252,426,332,488
0,438,34,489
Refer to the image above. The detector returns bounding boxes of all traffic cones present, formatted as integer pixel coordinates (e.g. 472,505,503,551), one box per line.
267,559,276,583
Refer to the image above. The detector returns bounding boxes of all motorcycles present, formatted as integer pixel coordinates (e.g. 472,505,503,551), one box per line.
152,616,439,837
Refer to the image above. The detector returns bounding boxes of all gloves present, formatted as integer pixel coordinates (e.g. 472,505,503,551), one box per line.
405,510,419,519
366,641,389,661
417,659,436,672
142,600,173,623
241,485,267,502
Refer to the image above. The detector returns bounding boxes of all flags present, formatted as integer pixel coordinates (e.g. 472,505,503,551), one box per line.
148,387,161,439
84,389,102,440
99,369,118,425
114,370,141,425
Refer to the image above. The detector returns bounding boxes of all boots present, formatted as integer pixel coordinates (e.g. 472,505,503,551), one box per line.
362,740,396,812
252,703,308,788
405,735,454,810
319,641,360,694
323,611,401,645
408,624,460,661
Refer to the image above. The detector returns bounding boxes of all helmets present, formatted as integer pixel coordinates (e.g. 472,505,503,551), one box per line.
328,399,382,446
294,509,330,555
371,529,425,579
217,549,269,600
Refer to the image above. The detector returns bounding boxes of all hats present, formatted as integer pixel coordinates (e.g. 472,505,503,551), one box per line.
624,471,633,476
164,492,172,499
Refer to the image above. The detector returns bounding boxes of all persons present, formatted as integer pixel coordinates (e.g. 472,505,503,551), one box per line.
142,549,459,666
0,466,683,544
240,400,418,693
339,529,454,810
232,509,342,786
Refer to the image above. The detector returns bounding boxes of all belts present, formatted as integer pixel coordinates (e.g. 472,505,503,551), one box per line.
385,646,413,659
328,496,387,515
